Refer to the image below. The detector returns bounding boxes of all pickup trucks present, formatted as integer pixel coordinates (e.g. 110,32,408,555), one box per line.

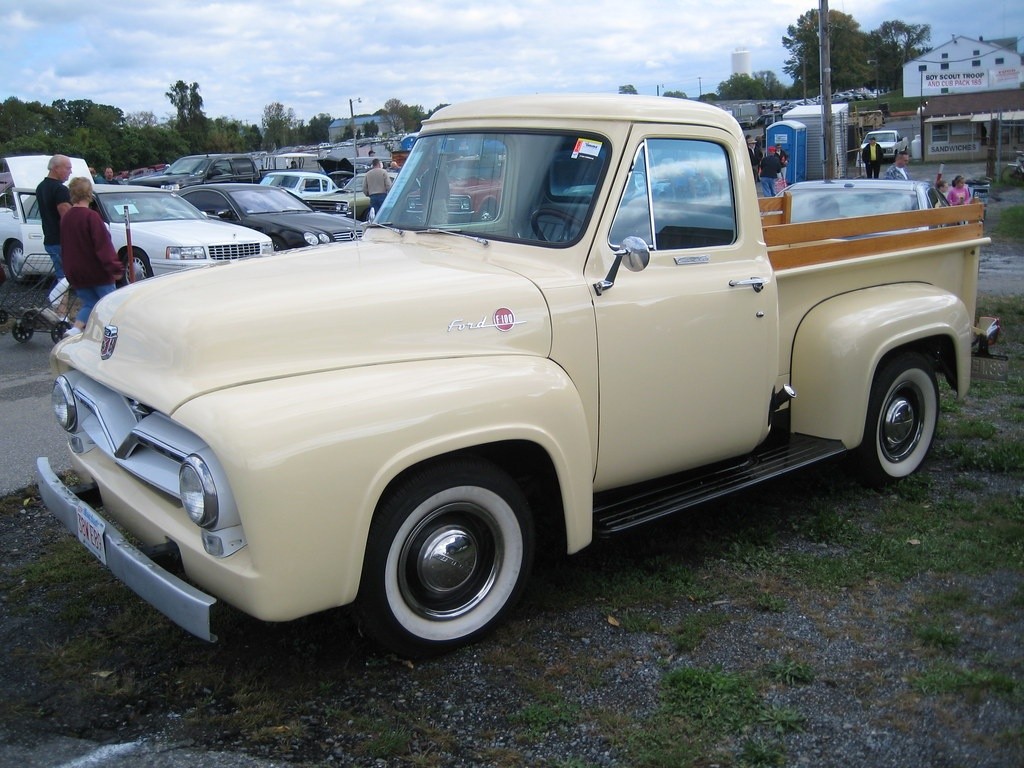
35,92,992,664
860,129,909,163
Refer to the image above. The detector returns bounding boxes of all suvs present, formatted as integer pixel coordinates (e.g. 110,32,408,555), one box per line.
124,153,263,196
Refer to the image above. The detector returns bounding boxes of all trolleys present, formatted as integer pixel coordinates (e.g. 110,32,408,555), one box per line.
0,253,80,345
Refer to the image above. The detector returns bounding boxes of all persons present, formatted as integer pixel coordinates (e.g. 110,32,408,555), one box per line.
59,176,124,338
36,154,74,323
745,134,969,224
290,151,450,226
88,168,129,185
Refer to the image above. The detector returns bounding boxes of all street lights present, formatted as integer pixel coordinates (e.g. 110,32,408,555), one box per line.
867,60,880,103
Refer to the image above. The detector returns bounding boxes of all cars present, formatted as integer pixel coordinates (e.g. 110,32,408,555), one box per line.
116,132,422,225
762,178,966,244
716,85,886,131
162,182,366,253
1,154,274,296
